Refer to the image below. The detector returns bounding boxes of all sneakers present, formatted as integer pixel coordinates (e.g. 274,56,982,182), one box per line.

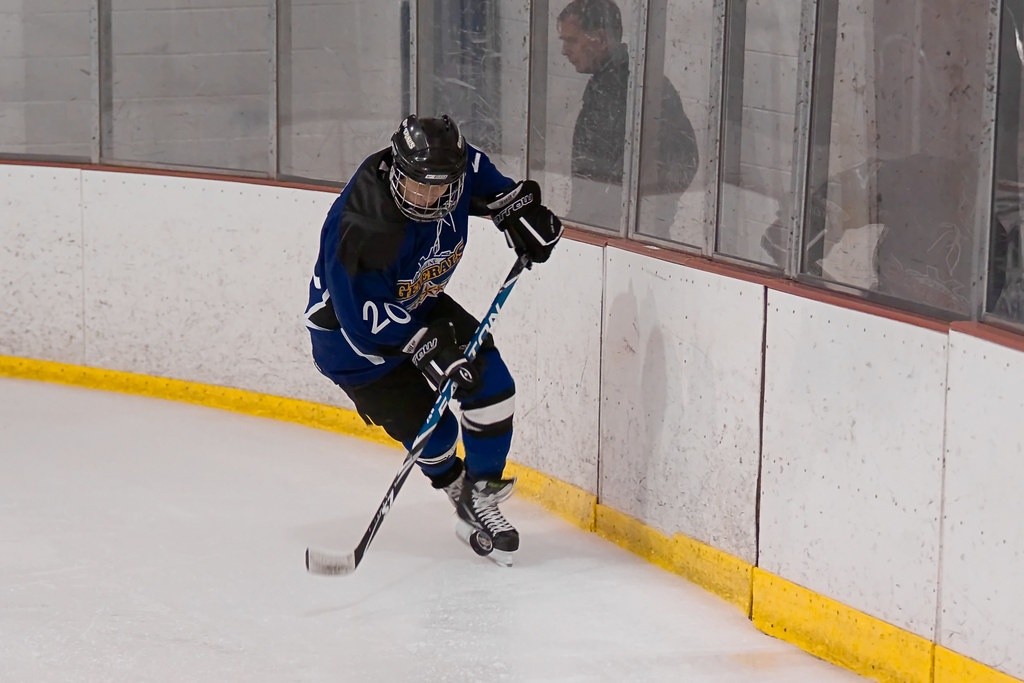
456,458,519,564
422,456,517,510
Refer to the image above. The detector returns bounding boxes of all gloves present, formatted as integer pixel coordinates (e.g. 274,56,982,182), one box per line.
485,180,564,271
399,319,478,399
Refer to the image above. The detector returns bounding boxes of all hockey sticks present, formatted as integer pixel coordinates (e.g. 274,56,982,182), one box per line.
305,250,527,578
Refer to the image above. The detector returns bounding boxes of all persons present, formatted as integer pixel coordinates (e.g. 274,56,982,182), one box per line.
762,150,1012,320
303,112,567,567
556,0,700,241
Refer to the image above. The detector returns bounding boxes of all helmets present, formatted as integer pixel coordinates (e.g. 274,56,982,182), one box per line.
390,114,469,217
